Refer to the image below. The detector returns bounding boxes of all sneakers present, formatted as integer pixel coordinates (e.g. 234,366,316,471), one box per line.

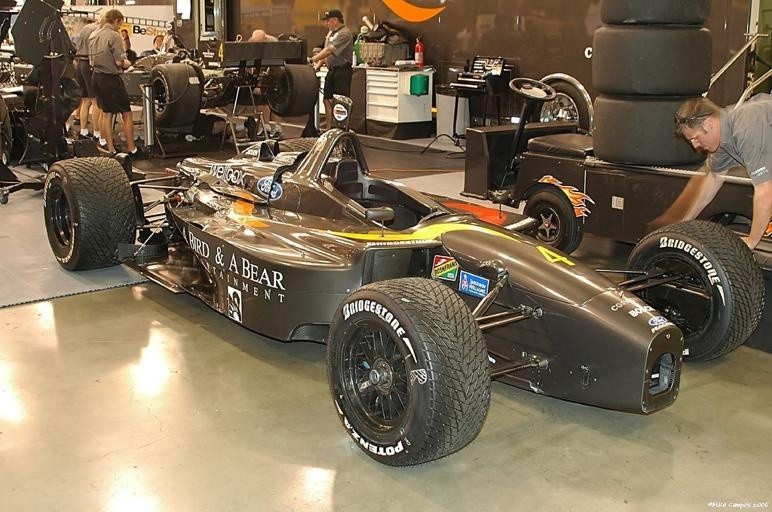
70,117,148,161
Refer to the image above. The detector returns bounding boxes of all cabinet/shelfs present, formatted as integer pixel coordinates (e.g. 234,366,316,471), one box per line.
345,66,366,136
316,64,331,124
365,66,432,138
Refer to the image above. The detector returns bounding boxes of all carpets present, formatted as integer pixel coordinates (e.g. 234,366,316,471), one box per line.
0,101,467,316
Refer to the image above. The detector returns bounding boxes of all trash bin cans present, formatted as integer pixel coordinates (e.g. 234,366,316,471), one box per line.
434,84,470,143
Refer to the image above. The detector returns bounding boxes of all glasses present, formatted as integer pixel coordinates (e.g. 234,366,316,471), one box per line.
674,112,713,124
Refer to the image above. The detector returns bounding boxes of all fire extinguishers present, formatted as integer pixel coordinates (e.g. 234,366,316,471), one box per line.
415,35,424,67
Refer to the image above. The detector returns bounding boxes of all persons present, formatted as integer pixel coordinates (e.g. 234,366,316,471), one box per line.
644,95,771,252
138,35,165,59
247,29,288,98
68,9,146,160
304,8,356,135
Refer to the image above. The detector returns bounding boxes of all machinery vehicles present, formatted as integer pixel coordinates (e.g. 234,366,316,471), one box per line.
460,73,771,276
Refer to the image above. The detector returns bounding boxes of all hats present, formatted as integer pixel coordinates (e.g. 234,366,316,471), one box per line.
320,10,342,20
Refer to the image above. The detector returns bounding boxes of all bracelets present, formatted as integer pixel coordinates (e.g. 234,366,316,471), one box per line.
309,57,313,64
